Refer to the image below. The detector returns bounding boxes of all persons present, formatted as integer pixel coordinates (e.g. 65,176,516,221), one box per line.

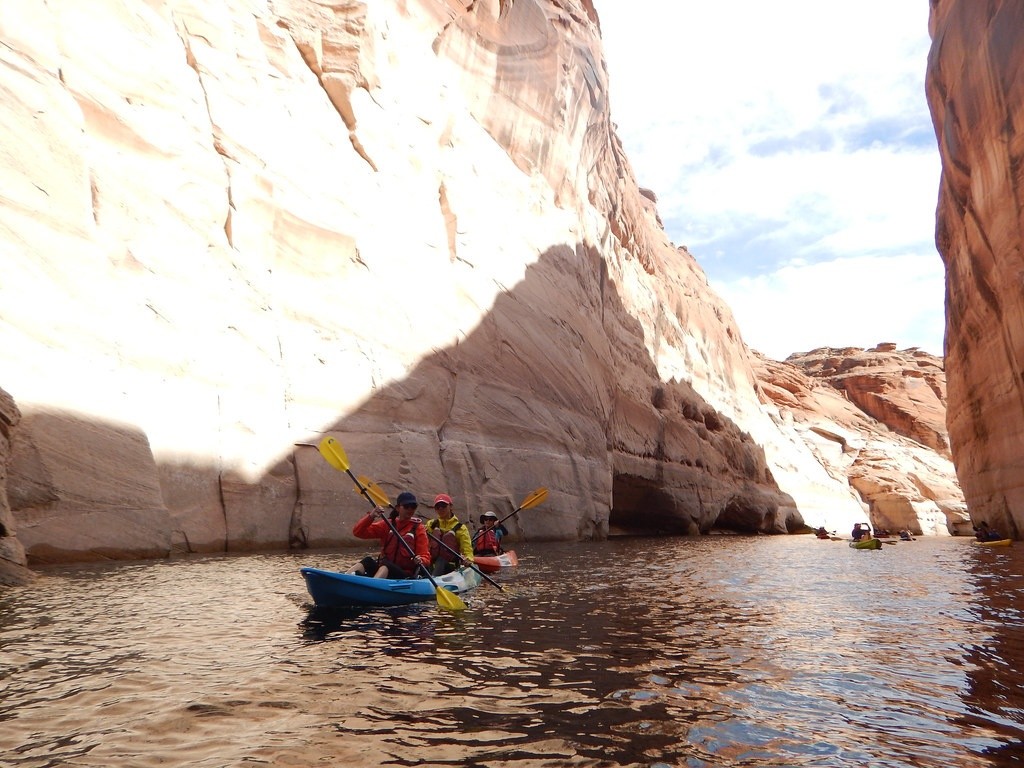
345,492,431,579
852,523,871,541
420,494,474,578
471,511,508,558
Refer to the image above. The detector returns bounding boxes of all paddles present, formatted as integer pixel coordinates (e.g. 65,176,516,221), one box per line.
353,474,509,592
318,435,469,610
471,486,549,542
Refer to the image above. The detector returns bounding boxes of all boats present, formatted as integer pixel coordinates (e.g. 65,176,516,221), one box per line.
850,533,883,549
972,538,1012,547
473,550,519,572
873,534,890,538
817,534,829,539
901,536,913,540
300,563,483,609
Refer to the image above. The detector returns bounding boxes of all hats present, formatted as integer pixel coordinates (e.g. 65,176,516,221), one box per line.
397,492,417,505
434,492,453,508
480,511,499,524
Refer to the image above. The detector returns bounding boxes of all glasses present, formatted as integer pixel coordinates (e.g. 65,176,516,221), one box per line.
434,502,447,510
484,517,492,521
403,503,417,510
